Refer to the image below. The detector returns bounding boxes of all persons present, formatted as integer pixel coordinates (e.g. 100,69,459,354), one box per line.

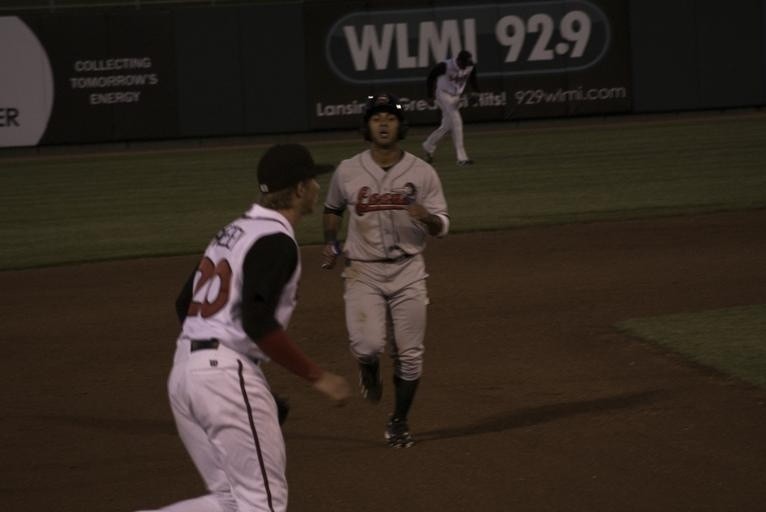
321,95,454,452
147,140,352,511
423,49,479,168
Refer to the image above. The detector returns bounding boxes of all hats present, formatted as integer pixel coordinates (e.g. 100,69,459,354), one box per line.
457,50,476,66
256,142,335,196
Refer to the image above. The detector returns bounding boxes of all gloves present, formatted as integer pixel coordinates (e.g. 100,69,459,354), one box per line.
308,367,356,410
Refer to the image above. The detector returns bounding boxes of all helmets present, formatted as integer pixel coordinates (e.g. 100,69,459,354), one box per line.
360,95,411,142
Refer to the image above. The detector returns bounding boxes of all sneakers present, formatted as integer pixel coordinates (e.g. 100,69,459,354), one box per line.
385,411,415,451
418,145,476,168
356,354,385,404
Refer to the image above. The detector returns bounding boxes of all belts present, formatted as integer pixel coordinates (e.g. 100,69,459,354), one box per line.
189,335,260,368
